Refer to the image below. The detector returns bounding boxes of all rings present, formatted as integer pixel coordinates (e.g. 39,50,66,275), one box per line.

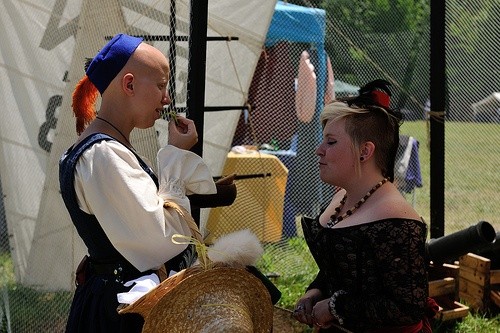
294,309,298,312
298,306,303,310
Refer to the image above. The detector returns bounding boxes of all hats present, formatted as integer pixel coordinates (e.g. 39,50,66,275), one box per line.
85,33,144,97
141,266,275,333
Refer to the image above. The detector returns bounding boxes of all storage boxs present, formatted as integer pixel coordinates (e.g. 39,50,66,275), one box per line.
428,250,500,327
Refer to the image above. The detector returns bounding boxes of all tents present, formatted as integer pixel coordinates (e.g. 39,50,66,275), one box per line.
471,91,500,123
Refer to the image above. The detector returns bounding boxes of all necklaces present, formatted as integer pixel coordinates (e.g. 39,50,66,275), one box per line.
325,176,391,228
96,117,138,151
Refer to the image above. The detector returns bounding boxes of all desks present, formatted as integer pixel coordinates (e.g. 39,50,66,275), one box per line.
392,134,423,211
255,147,299,239
203,144,289,246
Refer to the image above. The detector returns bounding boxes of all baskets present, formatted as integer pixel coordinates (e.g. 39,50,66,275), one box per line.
116,201,244,321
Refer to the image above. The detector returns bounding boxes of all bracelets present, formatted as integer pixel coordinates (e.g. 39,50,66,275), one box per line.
329,290,347,325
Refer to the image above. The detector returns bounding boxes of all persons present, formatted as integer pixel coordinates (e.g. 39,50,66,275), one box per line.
59,34,217,333
293,79,439,333
